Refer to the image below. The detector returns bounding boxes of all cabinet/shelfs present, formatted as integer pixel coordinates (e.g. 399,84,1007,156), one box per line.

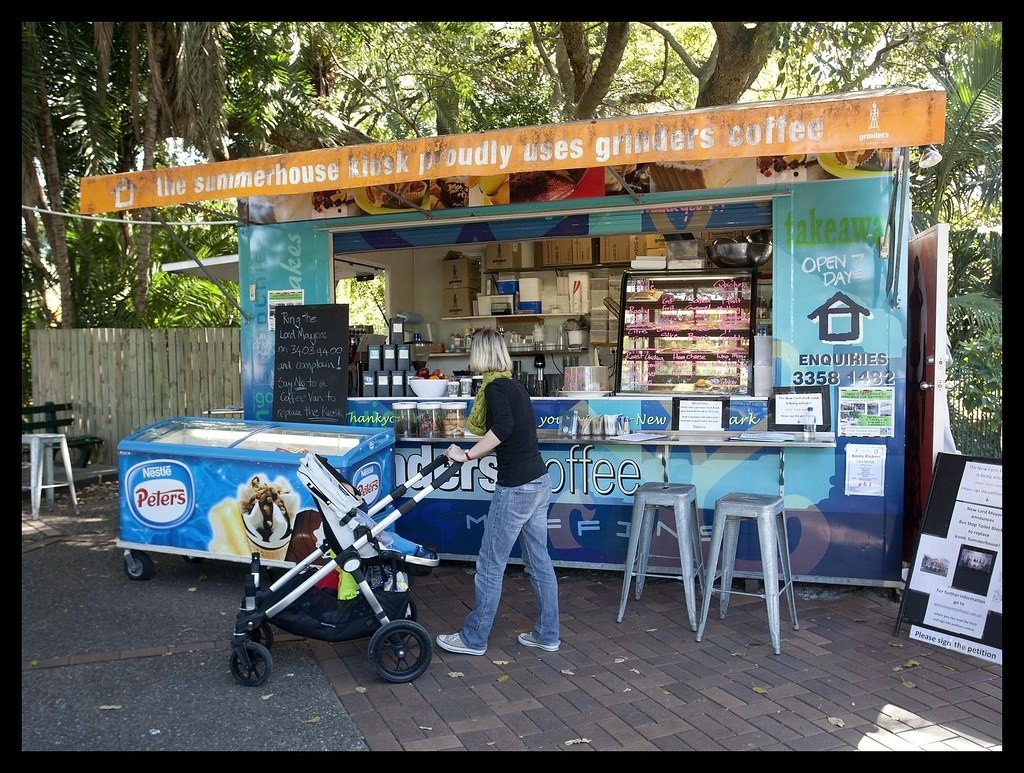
429,312,588,357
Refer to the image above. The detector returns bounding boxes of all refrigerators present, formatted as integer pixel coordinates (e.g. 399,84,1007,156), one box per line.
117,416,401,572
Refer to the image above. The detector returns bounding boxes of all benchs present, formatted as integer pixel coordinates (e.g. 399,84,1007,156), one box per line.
22,400,103,468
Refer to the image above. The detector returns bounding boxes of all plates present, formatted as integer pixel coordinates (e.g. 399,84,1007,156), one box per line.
816,153,897,179
526,168,589,201
558,390,612,398
352,188,431,214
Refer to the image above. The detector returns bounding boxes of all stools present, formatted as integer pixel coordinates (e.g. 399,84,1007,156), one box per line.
695,492,799,655
617,481,705,632
22,432,81,520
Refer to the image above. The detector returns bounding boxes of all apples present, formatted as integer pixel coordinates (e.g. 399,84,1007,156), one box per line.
417,367,448,379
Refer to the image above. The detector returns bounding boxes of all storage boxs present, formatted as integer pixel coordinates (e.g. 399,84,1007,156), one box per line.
589,275,621,343
631,255,708,269
629,234,648,262
442,288,474,317
520,300,542,313
496,279,518,295
361,317,416,397
485,242,523,269
645,233,668,257
441,250,481,289
665,239,706,259
533,237,593,267
592,236,631,264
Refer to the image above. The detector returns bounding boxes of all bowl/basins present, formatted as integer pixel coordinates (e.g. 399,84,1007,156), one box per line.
409,379,449,397
666,240,706,258
704,243,773,267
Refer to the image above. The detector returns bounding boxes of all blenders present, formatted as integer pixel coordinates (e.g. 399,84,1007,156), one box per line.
404,342,434,377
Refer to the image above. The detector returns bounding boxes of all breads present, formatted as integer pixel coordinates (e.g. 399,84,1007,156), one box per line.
696,379,705,387
649,157,749,190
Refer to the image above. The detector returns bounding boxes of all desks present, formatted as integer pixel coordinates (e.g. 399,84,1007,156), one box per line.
401,429,836,492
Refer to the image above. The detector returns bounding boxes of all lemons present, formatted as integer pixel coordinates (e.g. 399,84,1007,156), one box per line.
330,192,346,203
469,176,510,206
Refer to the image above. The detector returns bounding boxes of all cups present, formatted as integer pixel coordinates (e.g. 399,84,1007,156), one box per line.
591,415,603,436
471,376,484,395
460,378,472,396
579,419,590,435
604,415,617,435
447,381,459,398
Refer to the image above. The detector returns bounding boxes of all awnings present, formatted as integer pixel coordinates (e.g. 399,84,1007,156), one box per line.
79,84,946,318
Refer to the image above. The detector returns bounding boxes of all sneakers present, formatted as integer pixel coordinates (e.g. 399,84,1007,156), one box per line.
436,633,487,655
518,633,559,651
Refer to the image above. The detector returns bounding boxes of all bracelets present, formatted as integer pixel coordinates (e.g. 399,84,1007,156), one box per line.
465,453,472,460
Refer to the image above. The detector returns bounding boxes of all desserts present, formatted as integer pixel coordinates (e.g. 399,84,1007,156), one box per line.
366,181,427,209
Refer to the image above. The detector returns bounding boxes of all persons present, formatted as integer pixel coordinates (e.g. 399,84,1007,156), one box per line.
436,331,561,656
342,482,368,514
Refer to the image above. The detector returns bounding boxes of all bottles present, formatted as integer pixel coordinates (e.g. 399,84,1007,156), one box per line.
803,407,817,442
554,325,568,350
624,416,630,435
618,413,624,436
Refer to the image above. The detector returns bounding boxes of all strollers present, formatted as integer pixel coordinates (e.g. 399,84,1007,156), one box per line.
228,452,465,688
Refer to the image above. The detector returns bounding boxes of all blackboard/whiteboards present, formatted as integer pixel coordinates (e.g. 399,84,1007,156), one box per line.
899,452,1006,650
272,303,350,426
